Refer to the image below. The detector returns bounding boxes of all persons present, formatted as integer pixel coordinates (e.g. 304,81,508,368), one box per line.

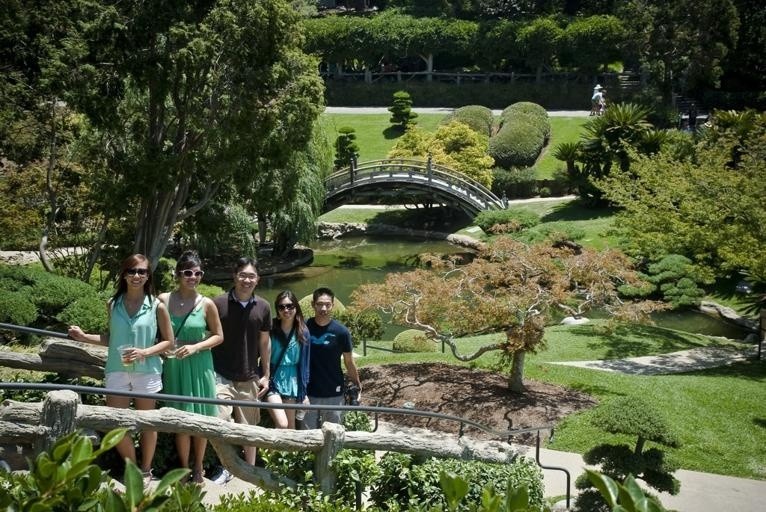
157,249,224,489
204,256,272,483
590,84,607,116
297,286,363,484
685,104,697,127
257,292,311,454
68,253,175,489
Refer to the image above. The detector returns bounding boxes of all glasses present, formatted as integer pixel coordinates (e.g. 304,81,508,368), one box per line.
127,268,148,277
277,304,296,310
179,269,204,279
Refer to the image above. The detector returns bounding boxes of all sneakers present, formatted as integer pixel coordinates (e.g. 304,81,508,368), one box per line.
213,468,233,486
181,469,205,487
141,470,153,489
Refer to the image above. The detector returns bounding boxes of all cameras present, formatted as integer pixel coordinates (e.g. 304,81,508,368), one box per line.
344,382,360,406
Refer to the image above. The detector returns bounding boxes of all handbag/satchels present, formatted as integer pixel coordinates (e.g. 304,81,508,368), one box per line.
258,367,276,391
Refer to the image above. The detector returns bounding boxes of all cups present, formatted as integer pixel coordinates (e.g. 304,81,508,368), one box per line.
295,409,306,421
165,338,178,359
116,345,135,367
